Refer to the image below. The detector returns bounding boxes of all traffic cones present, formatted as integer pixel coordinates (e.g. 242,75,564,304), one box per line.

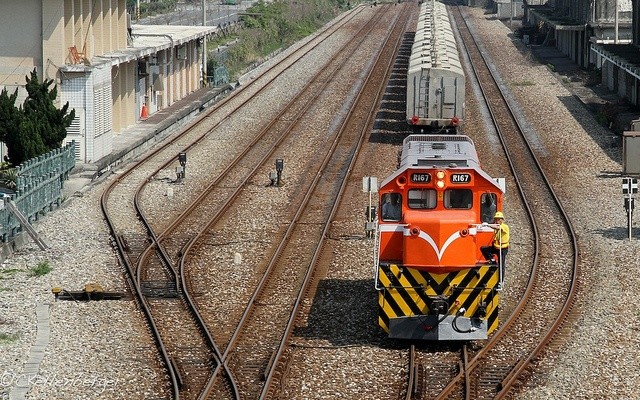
141,106,148,119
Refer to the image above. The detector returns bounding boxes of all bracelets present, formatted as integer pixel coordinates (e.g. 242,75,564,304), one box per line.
387,216,388,218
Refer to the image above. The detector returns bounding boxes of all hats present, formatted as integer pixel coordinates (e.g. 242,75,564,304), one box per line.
494,211,504,218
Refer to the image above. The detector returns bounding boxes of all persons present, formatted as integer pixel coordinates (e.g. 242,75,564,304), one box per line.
481,194,496,223
479,211,510,289
381,193,402,221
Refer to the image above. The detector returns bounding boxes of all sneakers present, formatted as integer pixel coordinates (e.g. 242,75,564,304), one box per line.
484,259,496,265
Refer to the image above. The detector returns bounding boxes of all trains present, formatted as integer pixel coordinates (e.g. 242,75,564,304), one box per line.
374,0,506,341
